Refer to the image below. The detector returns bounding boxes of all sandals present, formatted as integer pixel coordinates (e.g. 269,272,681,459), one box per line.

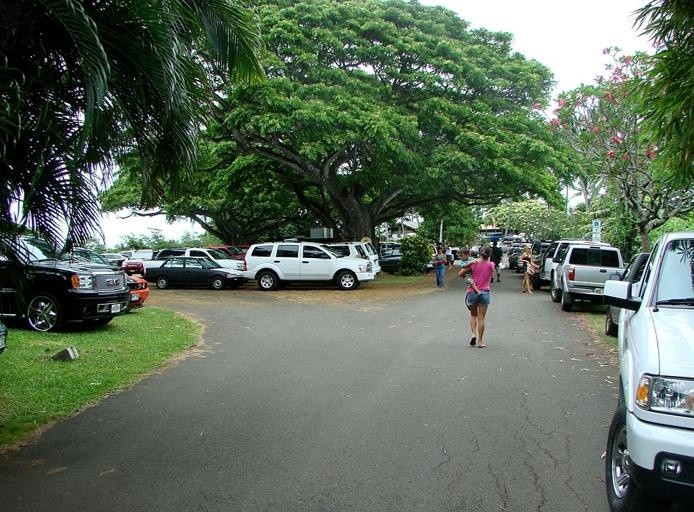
469,336,477,346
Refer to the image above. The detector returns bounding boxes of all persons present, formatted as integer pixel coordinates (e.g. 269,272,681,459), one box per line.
445,243,452,271
489,242,503,283
434,246,446,288
521,245,539,295
458,244,495,348
460,247,481,296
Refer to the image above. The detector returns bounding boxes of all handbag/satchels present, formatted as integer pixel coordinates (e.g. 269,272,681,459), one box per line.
527,265,535,275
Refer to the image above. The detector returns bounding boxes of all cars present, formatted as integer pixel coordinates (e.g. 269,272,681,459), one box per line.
63,247,150,307
0,320,9,355
470,232,622,313
602,252,651,338
323,241,467,275
143,256,249,290
100,243,251,291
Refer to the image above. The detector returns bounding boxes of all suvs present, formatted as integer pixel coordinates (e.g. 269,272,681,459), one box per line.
0,230,132,331
242,241,376,291
598,233,694,511
